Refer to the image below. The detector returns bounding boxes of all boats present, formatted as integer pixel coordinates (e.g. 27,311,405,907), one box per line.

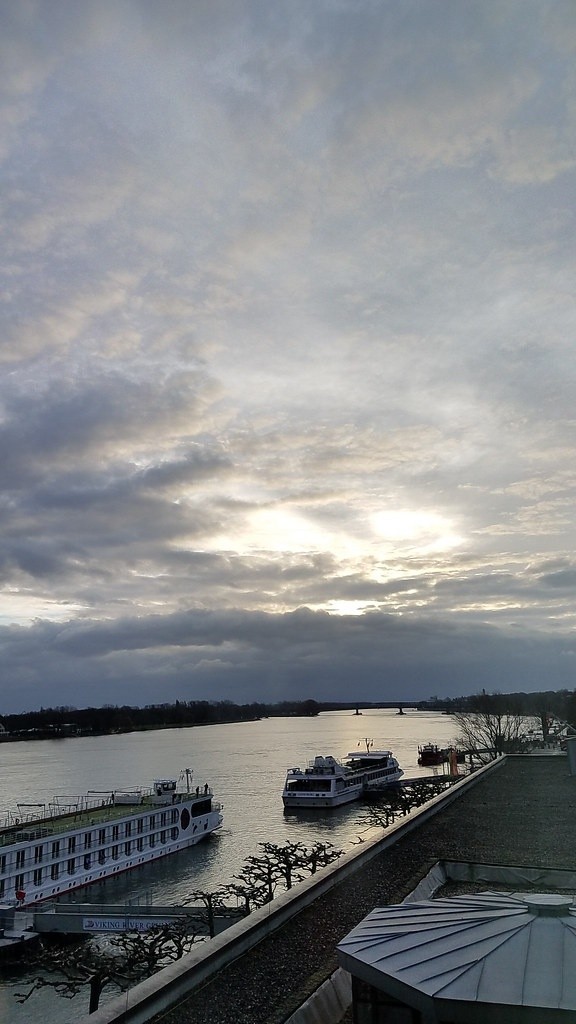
0,767,225,910
281,738,404,807
417,742,465,766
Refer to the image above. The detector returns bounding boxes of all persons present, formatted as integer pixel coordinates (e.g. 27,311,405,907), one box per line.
157,787,162,796
195,786,199,799
204,783,208,794
